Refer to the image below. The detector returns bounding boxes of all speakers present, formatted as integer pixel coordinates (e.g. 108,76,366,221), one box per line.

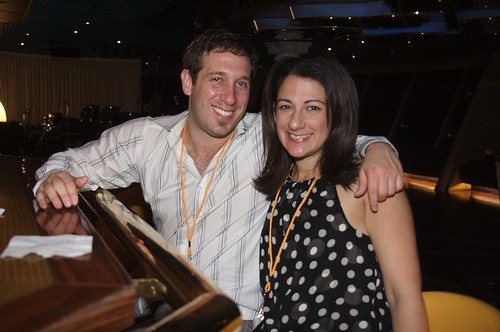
142,50,172,81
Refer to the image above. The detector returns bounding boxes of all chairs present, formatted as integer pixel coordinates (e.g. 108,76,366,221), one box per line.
421,291,500,332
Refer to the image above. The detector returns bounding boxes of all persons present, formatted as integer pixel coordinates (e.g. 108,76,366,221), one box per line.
33,28,403,332
252,51,429,332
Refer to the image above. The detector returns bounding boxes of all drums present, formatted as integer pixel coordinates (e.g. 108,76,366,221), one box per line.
40,112,66,134
99,105,121,130
79,104,99,128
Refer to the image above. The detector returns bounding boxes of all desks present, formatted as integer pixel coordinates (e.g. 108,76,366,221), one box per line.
0,154,242,332
